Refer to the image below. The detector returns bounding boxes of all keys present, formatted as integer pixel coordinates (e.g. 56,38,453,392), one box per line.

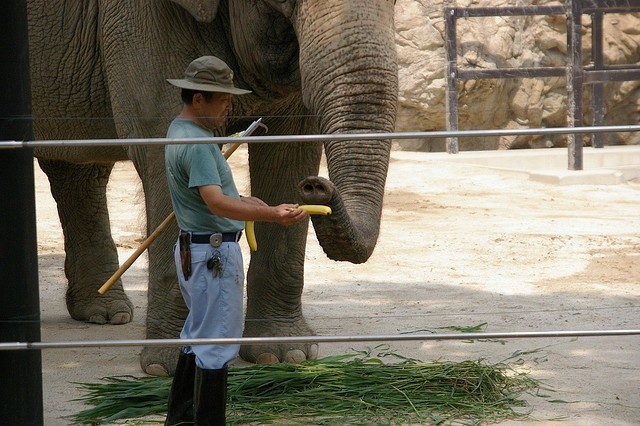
206,250,224,279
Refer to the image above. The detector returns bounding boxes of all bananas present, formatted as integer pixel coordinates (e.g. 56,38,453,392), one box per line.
289,204,332,218
244,220,258,251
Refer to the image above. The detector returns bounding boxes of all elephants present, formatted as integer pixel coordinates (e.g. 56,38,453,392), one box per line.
27,0,398,379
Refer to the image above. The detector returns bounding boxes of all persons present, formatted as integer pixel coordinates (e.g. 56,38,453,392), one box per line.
164,55,308,426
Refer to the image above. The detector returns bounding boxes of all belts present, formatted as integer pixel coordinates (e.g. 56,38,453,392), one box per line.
192,232,242,243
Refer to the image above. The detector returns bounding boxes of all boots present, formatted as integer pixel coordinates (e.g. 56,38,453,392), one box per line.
192,365,228,426
165,353,196,426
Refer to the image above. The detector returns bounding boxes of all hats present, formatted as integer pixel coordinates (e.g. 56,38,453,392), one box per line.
165,55,253,95
